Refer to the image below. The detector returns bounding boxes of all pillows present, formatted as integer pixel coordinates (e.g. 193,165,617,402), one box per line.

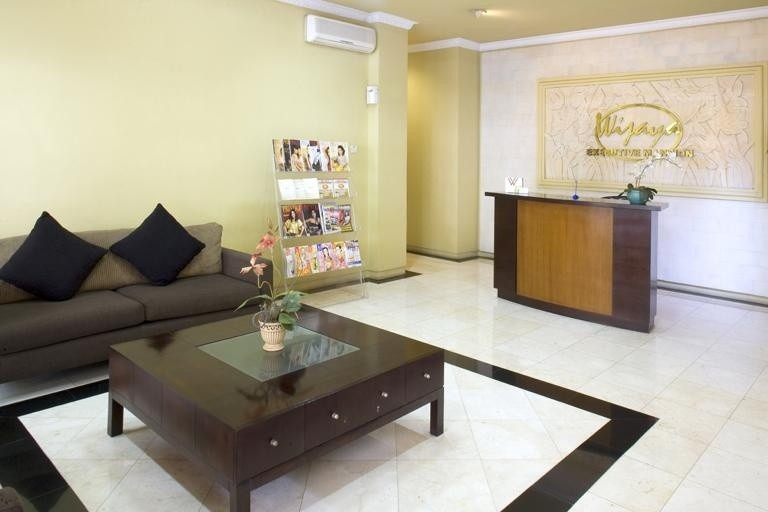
0,203,223,302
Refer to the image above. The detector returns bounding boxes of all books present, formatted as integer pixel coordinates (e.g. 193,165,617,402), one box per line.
273,139,363,280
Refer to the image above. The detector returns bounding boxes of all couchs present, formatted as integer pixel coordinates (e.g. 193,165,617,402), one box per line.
1,222,273,384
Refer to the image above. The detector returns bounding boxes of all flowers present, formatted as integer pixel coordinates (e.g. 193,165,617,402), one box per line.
234,219,312,330
624,151,682,199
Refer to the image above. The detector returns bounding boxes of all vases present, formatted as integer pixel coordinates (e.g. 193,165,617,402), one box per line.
256,320,286,351
626,190,649,205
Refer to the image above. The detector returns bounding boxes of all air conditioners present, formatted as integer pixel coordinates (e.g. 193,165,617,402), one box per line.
304,14,376,53
